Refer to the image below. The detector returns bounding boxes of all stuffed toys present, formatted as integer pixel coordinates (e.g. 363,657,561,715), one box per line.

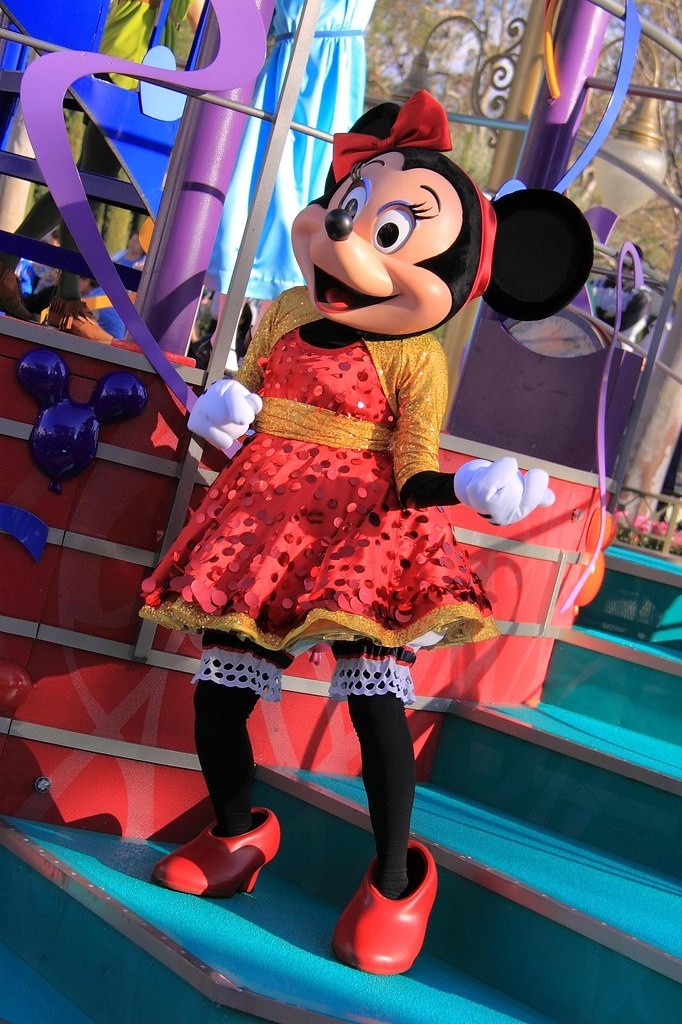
140,101,594,976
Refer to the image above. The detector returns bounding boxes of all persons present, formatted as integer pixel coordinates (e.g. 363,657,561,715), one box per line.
588,242,651,345
14,230,127,341
111,231,147,271
185,303,253,371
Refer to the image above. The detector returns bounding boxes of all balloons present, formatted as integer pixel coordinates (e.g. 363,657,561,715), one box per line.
15,347,149,495
